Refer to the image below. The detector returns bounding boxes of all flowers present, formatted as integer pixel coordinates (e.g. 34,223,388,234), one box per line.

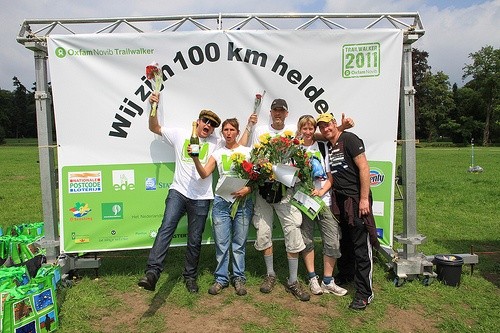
232,133,312,205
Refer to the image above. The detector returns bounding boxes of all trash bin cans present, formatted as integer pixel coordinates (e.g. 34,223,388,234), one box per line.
433,253,464,288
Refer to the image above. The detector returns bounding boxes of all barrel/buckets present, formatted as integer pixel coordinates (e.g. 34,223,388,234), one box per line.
435,254,464,287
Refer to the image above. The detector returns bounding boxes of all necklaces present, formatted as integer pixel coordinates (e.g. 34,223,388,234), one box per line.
226,143,237,152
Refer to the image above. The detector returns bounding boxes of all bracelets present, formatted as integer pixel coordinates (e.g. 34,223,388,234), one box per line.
150,105,159,110
246,125,253,132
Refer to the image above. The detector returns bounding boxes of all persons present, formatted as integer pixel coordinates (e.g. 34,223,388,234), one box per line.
287,114,350,297
315,112,381,310
251,98,356,301
186,117,259,296
136,91,258,295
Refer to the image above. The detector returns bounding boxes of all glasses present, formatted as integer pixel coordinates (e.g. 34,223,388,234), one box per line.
200,117,217,129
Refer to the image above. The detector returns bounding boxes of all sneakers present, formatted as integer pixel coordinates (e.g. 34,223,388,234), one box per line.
183,277,198,294
286,280,310,302
208,282,224,295
137,271,158,292
349,291,374,311
234,278,247,296
320,276,348,296
260,272,277,294
308,276,322,295
333,274,352,285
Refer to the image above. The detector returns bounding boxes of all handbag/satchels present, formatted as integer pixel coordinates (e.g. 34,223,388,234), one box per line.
9,235,27,265
20,235,48,262
0,234,12,260
28,220,46,239
0,265,29,332
2,284,59,333
35,264,61,295
6,222,29,235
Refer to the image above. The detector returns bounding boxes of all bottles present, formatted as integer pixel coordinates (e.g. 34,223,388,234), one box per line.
190,121,199,157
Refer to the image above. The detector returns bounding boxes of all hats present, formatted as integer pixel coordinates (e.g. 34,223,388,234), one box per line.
198,109,221,128
271,98,288,112
316,114,333,125
